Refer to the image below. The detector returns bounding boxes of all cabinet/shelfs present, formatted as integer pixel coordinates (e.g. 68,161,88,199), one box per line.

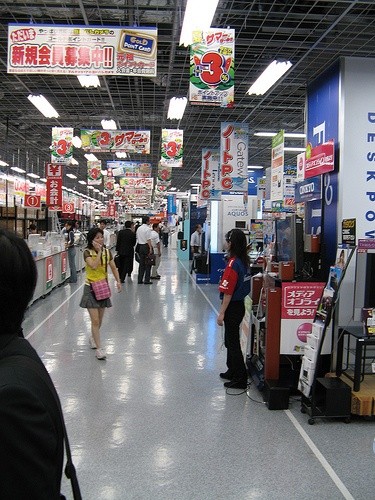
249,260,334,392
297,247,357,407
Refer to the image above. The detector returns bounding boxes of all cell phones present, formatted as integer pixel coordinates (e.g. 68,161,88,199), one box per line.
91,240,102,248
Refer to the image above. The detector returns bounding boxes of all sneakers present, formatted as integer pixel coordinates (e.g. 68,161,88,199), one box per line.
89,336,97,349
96,348,106,360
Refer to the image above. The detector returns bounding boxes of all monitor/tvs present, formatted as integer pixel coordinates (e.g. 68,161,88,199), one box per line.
275,214,304,273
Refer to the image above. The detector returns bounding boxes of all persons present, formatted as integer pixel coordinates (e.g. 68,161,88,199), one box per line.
99,220,110,249
0,228,70,500
217,229,252,389
94,220,110,231
65,222,78,283
161,222,169,247
115,221,125,235
136,216,154,284
335,250,345,268
158,221,164,239
79,227,122,360
27,224,37,240
61,219,81,234
149,223,161,279
190,224,202,274
130,221,141,234
115,221,137,283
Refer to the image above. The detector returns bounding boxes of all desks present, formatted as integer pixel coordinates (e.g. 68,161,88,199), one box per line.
335,325,375,392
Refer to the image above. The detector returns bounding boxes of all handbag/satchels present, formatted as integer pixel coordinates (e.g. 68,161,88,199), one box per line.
114,255,120,268
90,279,111,300
145,253,156,266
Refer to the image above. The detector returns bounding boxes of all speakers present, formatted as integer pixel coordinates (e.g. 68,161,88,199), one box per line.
262,379,290,410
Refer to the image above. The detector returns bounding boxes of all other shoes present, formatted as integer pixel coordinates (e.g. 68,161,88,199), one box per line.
144,282,153,284
67,279,72,283
151,277,160,279
128,274,131,276
156,275,161,277
121,279,125,283
138,282,144,284
224,380,247,389
220,371,235,380
